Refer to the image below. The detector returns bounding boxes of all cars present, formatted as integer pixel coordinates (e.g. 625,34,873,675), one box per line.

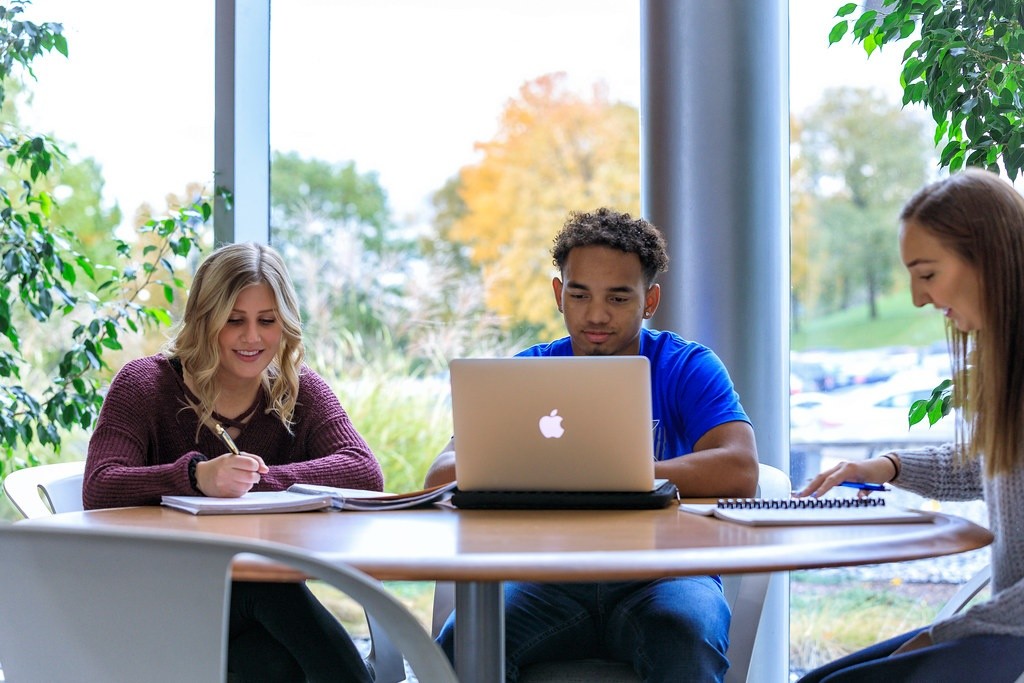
787,345,964,439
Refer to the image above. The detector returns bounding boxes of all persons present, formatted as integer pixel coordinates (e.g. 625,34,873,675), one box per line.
795,165,1023,683
83,242,384,683
424,209,758,682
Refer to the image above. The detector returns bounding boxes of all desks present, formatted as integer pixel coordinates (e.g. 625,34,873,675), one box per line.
12,504,999,683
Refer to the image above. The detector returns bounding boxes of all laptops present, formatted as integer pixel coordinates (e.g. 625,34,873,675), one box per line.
449,355,669,492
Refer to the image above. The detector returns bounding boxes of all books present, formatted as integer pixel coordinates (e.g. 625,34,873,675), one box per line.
161,480,458,516
678,497,935,526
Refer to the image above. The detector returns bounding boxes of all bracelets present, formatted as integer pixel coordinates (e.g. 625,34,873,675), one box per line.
188,454,206,497
880,455,898,483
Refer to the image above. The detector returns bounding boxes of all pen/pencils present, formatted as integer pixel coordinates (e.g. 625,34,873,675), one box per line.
213,422,237,454
810,476,892,492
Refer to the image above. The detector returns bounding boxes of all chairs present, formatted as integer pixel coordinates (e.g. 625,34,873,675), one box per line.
1,520,459,682
431,461,793,682
5,462,406,683
